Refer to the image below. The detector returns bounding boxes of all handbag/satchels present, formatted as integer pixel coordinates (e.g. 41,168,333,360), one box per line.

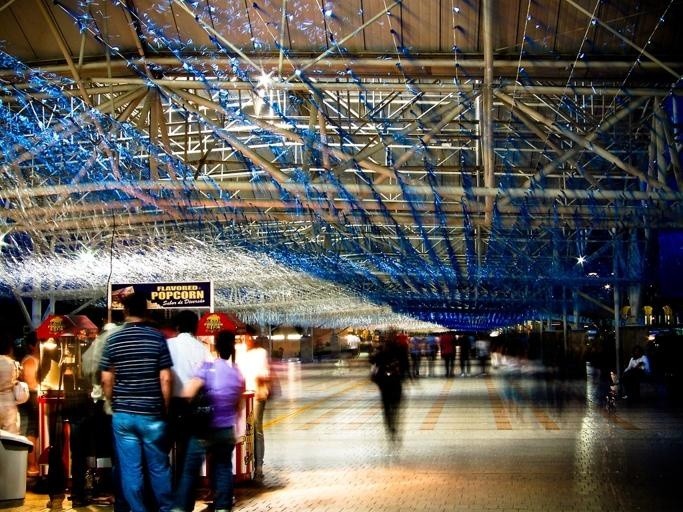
195,396,213,424
13,382,30,405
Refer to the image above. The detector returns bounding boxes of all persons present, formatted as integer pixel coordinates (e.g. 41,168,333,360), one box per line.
2,291,286,511
368,333,414,445
345,330,499,377
501,329,683,417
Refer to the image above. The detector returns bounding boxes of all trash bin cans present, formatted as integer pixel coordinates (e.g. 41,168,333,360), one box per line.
0,430,33,509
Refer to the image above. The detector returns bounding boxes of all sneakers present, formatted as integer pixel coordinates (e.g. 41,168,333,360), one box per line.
252,472,263,484
